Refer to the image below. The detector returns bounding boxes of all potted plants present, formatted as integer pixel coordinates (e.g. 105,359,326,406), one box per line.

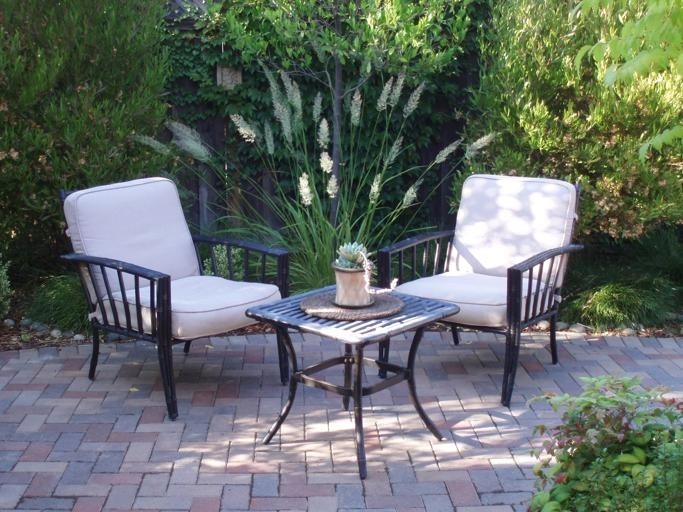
329,239,376,308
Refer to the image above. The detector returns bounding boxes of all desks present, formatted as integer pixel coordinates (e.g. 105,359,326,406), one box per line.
244,279,462,479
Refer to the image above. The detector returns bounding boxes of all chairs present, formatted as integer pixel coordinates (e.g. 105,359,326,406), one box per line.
59,175,292,420
379,173,586,409
